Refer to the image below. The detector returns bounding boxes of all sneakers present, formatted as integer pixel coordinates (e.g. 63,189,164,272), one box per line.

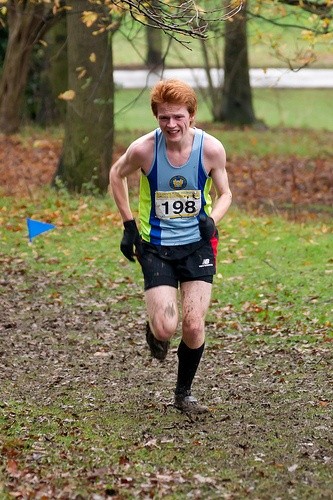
173,395,209,413
144,322,168,360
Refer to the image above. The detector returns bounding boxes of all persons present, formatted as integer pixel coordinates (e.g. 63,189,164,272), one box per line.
110,80,232,413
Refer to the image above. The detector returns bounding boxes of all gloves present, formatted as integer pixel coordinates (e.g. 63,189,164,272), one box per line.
198,217,215,241
120,220,144,263
